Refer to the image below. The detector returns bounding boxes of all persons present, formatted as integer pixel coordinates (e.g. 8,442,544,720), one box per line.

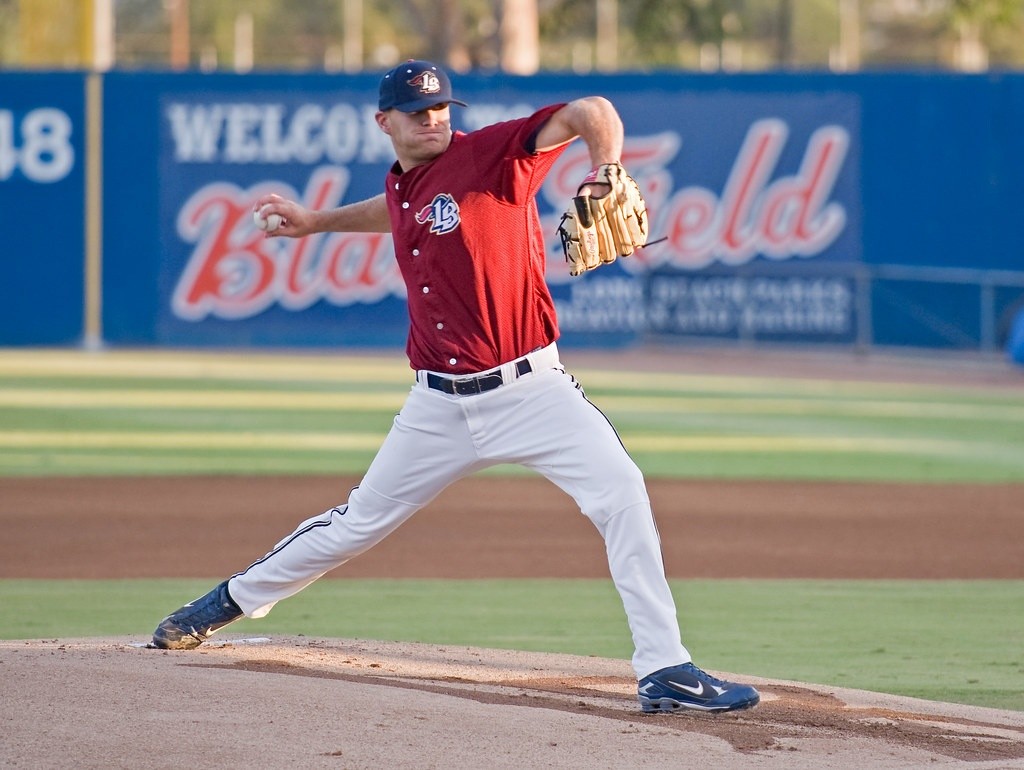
151,61,759,714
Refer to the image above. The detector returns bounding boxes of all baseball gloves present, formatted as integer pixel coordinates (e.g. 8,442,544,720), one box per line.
556,161,651,278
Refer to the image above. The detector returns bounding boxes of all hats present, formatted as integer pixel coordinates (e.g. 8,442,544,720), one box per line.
379,59,468,113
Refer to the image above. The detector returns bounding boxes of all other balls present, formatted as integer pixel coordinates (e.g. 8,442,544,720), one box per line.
252,202,283,233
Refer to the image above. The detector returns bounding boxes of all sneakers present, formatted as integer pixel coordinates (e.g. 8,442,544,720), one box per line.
153,580,244,650
638,661,760,716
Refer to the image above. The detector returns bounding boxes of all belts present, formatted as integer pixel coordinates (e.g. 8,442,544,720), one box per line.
416,359,532,397
